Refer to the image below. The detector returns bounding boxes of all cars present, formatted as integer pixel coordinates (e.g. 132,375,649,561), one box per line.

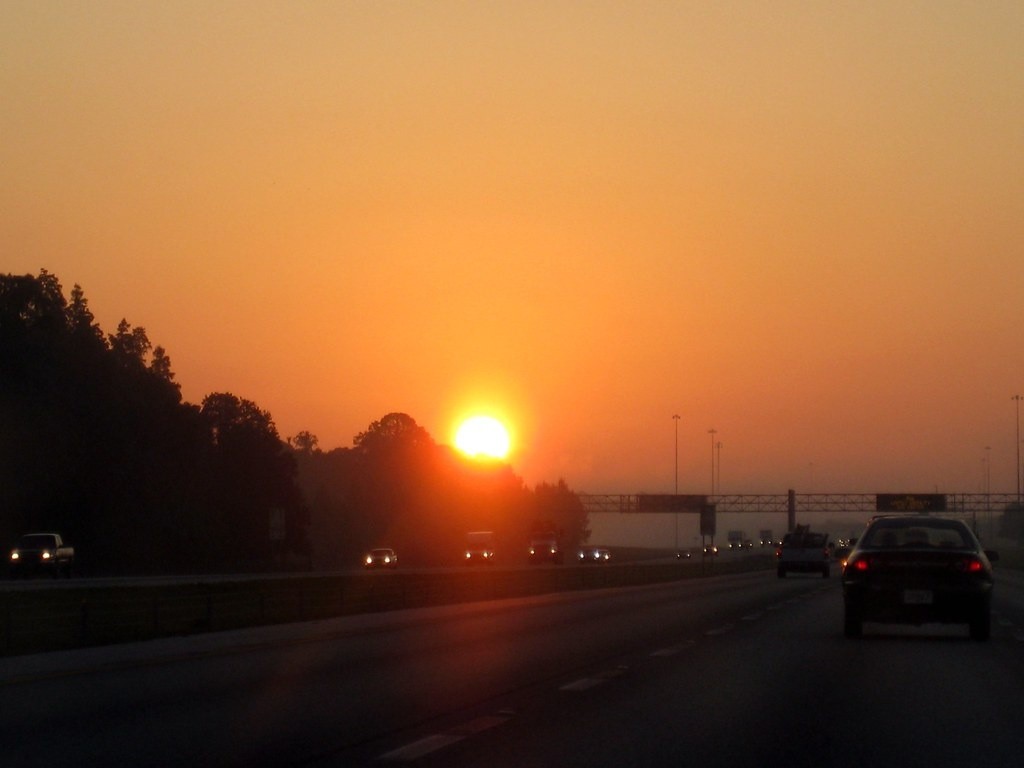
366,548,399,569
837,513,1000,641
577,531,836,580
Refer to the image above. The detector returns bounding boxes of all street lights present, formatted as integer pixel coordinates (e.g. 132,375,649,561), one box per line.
1011,394,1024,506
672,414,681,496
707,428,718,501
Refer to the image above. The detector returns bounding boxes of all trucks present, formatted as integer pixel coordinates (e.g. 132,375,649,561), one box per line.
462,530,497,567
527,532,566,565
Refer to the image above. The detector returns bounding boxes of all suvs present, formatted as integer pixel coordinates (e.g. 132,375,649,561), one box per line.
8,533,75,578
